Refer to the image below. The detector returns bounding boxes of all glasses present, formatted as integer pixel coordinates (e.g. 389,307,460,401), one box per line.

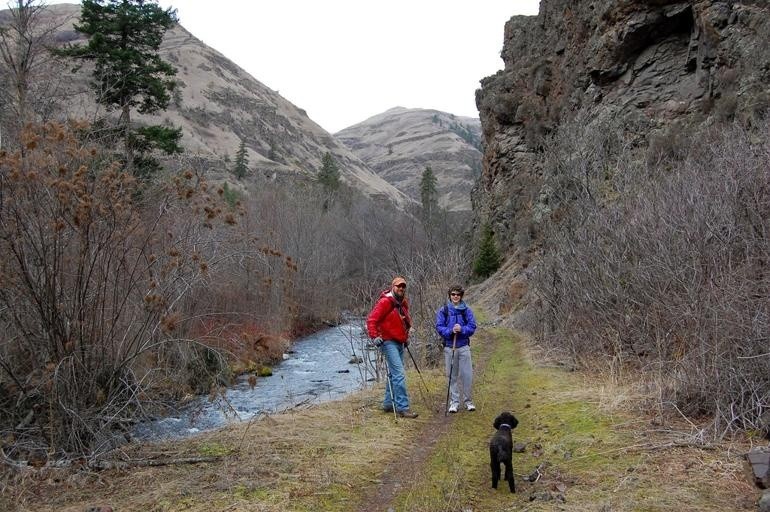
450,293,460,296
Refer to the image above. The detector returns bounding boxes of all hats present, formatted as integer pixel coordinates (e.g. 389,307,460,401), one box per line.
392,276,407,288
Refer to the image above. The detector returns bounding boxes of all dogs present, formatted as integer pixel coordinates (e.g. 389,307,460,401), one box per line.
489,411,519,494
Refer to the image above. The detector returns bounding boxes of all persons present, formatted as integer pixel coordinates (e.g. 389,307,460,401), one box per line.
366,277,419,419
434,284,479,413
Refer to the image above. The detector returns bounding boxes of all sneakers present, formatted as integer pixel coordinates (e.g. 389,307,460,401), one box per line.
382,406,418,418
463,401,476,411
448,399,460,413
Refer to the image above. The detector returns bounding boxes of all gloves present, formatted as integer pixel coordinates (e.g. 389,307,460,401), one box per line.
373,337,384,348
